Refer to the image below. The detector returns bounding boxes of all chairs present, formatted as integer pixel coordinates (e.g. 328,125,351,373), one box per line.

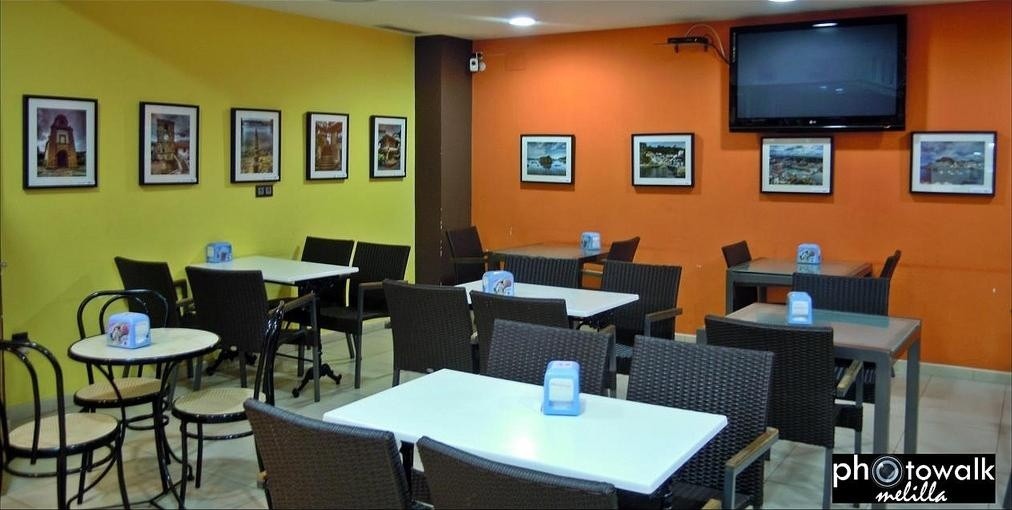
0,221,925,510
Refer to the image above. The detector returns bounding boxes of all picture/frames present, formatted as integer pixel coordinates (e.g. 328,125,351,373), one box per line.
758,127,836,199
302,109,353,184
905,130,1000,200
629,129,697,191
229,108,284,187
365,112,409,182
20,92,101,194
519,132,577,187
134,97,203,189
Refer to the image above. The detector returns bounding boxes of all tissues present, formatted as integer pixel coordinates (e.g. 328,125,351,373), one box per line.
205,242,233,264
481,270,516,300
107,312,151,350
543,360,580,415
786,292,813,326
579,231,601,251
796,243,823,264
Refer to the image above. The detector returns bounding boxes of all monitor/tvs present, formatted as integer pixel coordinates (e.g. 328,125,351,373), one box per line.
729,13,907,131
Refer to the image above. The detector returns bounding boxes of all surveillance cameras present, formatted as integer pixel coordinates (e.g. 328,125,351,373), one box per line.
470,57,479,72
